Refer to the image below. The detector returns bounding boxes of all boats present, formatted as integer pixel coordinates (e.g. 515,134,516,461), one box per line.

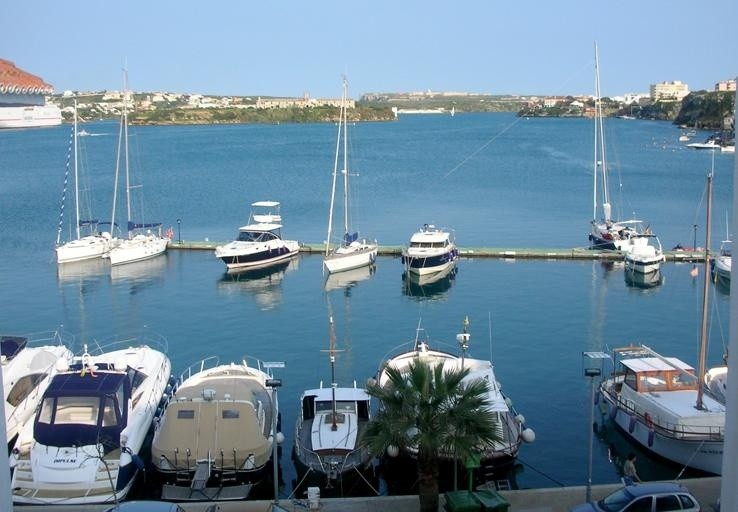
55,257,110,340
323,75,379,273
216,255,301,313
324,264,378,365
212,199,303,269
53,97,123,265
402,257,462,304
110,253,172,288
107,55,170,265
400,224,460,276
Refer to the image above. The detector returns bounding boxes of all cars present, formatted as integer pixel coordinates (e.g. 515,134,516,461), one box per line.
569,479,703,512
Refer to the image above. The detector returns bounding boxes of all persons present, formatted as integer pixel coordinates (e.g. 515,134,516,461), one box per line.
98,232,113,251
623,452,642,486
